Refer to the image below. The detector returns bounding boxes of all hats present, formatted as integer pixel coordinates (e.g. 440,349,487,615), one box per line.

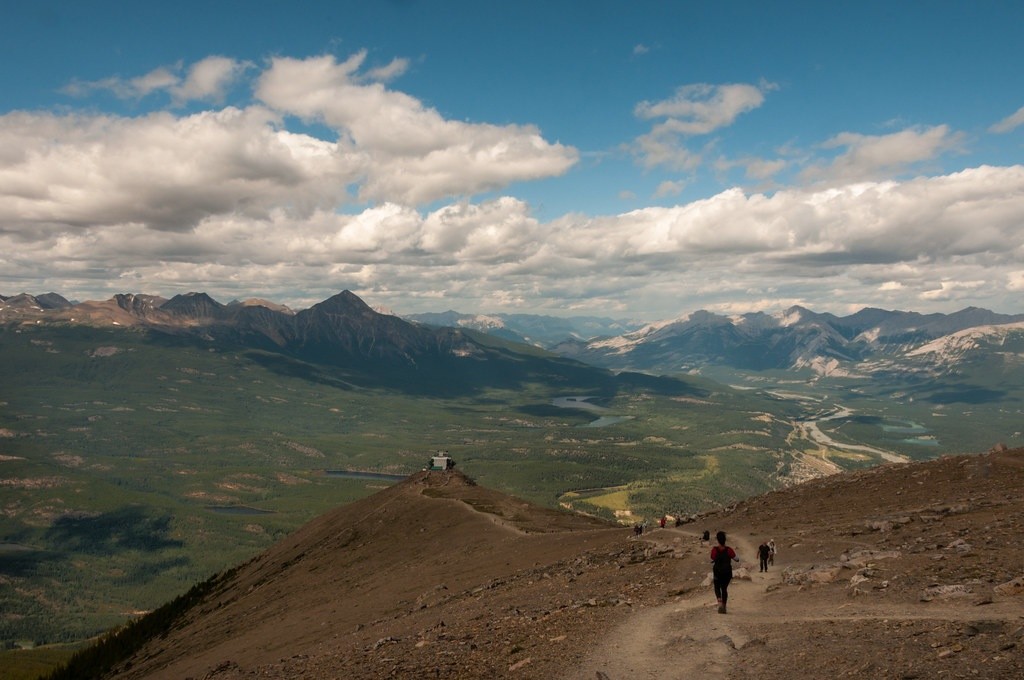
763,540,766,544
771,539,774,543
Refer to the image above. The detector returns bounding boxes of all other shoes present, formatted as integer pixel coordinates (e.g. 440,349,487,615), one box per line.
723,606,726,613
765,570,767,572
759,570,763,572
768,560,769,564
717,603,724,613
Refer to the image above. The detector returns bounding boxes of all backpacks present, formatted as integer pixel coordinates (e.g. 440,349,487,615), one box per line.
715,546,732,580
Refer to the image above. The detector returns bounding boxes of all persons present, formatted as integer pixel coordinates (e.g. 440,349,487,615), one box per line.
634,521,647,538
756,541,770,573
661,515,681,529
768,539,778,566
711,531,740,614
